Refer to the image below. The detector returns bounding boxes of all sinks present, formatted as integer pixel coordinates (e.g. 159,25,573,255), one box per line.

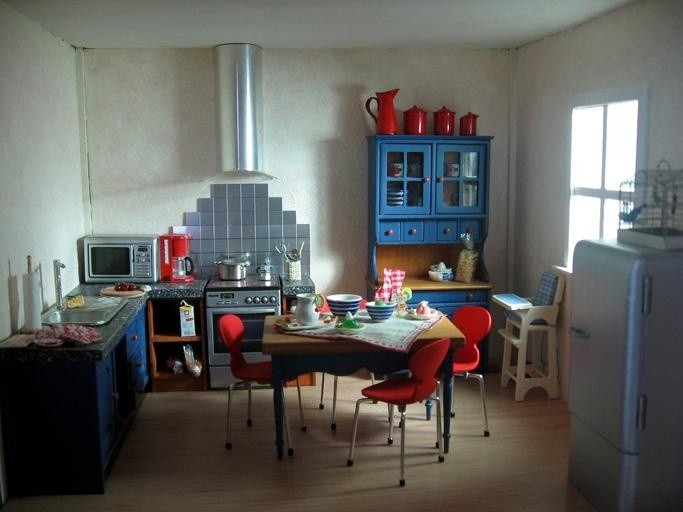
40,310,114,326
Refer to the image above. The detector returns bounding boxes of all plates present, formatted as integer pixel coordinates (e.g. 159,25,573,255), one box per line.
273,313,337,331
101,287,145,298
388,187,409,206
406,309,438,320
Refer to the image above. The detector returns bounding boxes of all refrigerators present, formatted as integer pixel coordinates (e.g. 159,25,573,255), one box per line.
564,238,683,512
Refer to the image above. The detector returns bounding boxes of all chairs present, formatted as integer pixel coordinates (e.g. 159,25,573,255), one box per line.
219,314,307,457
491,270,565,401
347,339,452,488
319,298,379,430
441,308,492,444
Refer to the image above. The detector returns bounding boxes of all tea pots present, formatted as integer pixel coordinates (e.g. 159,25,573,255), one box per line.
256,264,275,282
365,88,402,135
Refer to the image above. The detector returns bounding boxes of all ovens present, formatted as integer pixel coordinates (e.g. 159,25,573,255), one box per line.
206,306,283,390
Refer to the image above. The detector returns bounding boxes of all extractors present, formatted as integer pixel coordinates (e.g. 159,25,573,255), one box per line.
199,43,277,186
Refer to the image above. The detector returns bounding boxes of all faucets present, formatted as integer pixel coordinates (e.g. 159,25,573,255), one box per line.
52,260,66,311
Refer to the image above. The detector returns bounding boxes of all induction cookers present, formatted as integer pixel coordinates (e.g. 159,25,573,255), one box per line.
205,274,282,307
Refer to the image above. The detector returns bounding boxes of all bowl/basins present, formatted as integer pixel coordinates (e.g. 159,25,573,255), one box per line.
428,270,454,282
325,294,362,320
365,302,397,323
335,324,365,335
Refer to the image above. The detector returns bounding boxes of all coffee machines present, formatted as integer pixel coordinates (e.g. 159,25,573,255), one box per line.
160,235,197,284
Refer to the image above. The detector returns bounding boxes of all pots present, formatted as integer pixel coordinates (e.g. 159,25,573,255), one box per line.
212,254,252,282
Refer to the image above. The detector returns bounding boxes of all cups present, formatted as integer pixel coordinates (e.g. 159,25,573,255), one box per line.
447,164,459,177
295,292,325,326
390,163,409,177
284,261,302,282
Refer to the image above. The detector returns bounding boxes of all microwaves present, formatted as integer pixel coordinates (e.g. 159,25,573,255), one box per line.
82,235,159,284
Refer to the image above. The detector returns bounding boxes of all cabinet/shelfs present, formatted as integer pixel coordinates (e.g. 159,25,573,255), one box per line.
0,307,148,496
149,297,207,392
366,279,494,381
369,135,492,282
